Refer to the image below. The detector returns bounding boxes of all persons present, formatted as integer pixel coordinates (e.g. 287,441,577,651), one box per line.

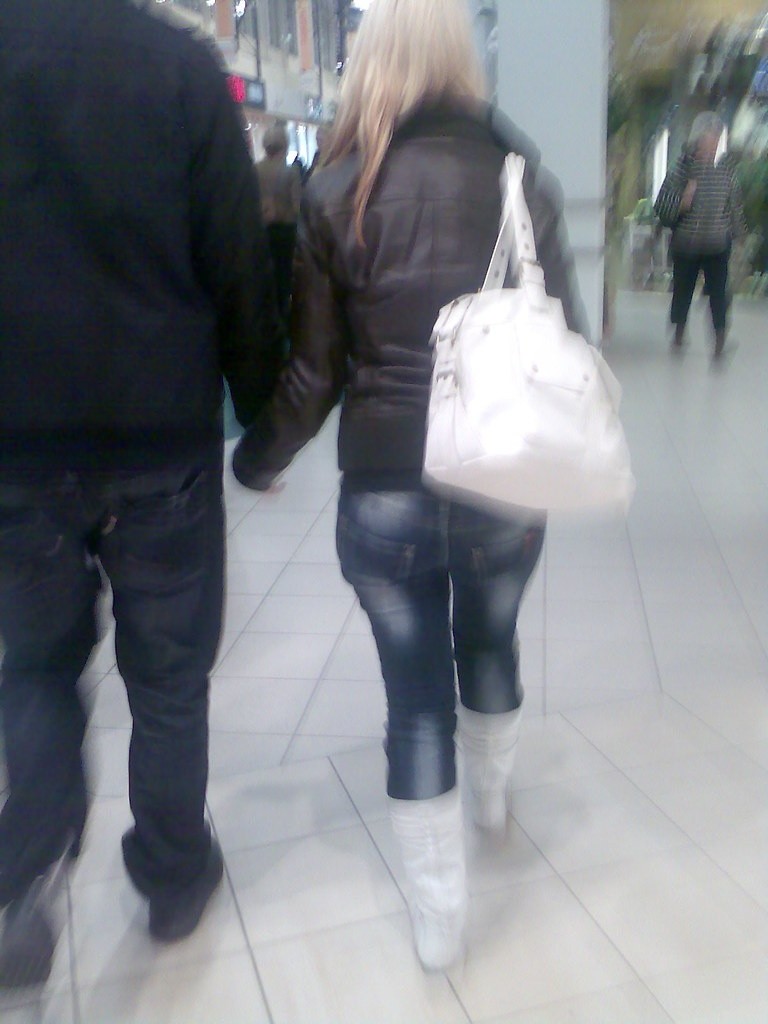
654,111,742,365
0,1,287,989
304,123,331,188
255,125,302,220
233,1,589,972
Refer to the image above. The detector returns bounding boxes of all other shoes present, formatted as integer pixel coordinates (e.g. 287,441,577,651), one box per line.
0,898,55,986
149,836,224,943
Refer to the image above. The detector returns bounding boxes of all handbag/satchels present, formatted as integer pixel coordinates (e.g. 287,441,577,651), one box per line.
424,153,633,512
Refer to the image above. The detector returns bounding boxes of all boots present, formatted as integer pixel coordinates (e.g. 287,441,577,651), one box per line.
455,685,526,831
386,784,467,969
